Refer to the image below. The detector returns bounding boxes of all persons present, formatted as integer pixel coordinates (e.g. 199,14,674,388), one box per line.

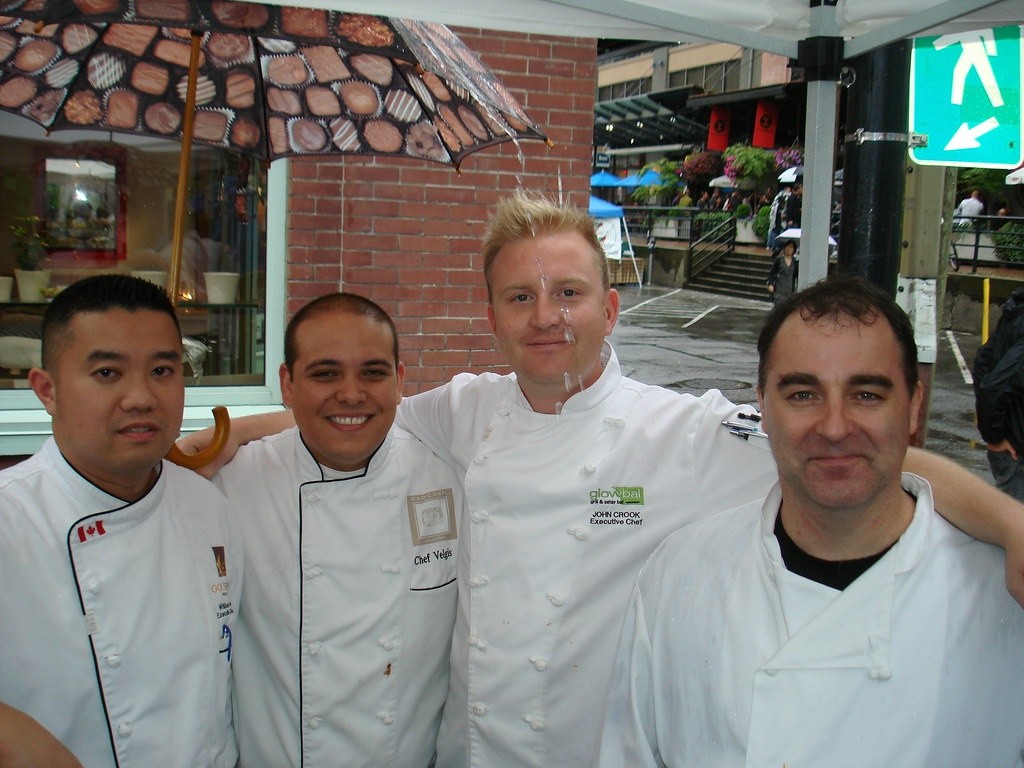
207,292,463,768
0,273,246,768
940,190,1011,232
597,277,1024,768
170,186,1024,768
671,184,843,308
974,285,1023,504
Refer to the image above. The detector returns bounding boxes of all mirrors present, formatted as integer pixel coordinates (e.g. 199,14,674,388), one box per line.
32,140,127,270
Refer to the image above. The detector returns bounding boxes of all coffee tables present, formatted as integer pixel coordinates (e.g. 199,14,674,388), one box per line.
0,298,51,314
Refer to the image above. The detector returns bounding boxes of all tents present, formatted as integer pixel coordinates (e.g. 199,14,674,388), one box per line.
589,195,643,288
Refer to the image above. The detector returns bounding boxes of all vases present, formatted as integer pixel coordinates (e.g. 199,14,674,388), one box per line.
0,276,13,303
131,271,168,291
203,272,239,304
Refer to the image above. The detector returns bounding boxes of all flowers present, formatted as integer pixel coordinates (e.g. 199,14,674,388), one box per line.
723,156,737,179
774,147,801,169
676,170,682,174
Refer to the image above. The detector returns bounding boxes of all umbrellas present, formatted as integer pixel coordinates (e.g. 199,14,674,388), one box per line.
708,175,737,188
1005,167,1024,184
735,175,766,216
0,0,554,468
590,169,671,189
773,228,838,248
778,166,804,182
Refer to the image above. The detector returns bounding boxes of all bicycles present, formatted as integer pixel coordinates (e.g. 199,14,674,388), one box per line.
950,244,960,271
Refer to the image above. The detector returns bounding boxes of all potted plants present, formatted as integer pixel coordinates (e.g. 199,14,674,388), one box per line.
630,158,691,240
684,152,736,242
9,216,51,304
722,143,774,243
